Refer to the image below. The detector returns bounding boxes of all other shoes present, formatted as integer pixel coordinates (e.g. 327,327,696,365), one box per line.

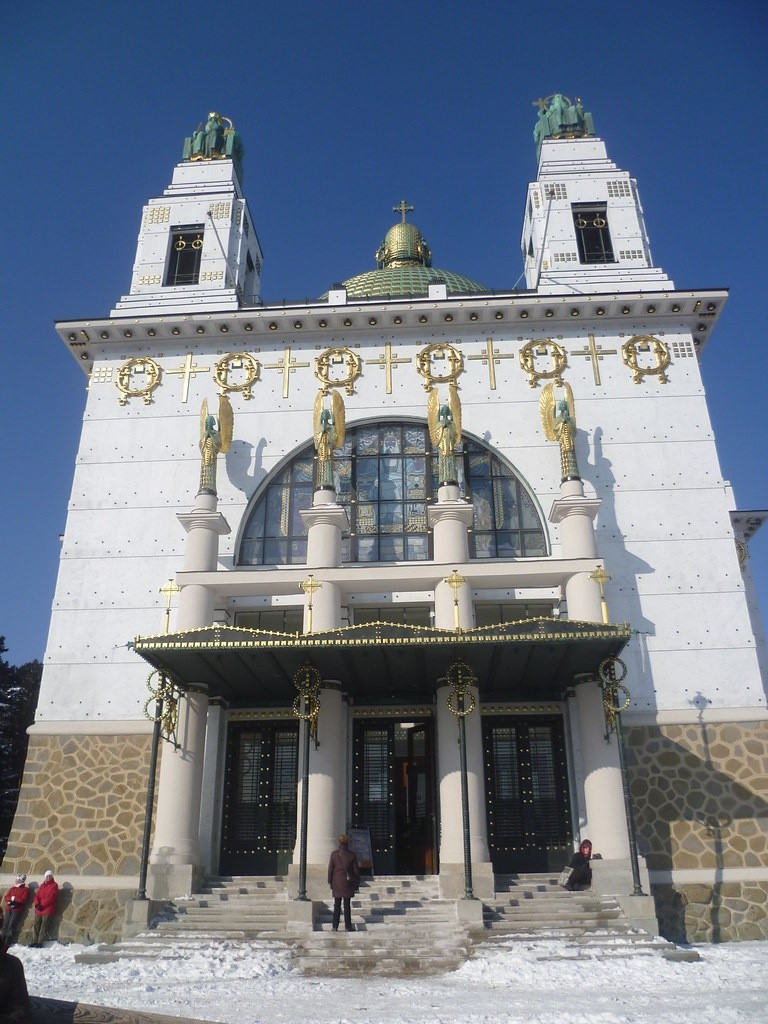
332,928,337,931
348,928,355,930
28,943,43,948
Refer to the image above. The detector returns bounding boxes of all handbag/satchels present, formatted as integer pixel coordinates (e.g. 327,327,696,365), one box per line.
558,865,574,891
347,873,361,886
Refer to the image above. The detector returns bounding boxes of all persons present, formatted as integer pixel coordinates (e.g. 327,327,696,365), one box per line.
328,834,360,931
28,870,59,947
564,840,592,892
1,874,29,944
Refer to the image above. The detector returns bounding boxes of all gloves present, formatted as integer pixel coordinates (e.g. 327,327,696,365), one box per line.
36,904,42,910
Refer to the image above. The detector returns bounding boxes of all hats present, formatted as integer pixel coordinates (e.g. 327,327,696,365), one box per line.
45,870,53,878
17,875,26,880
339,834,348,844
